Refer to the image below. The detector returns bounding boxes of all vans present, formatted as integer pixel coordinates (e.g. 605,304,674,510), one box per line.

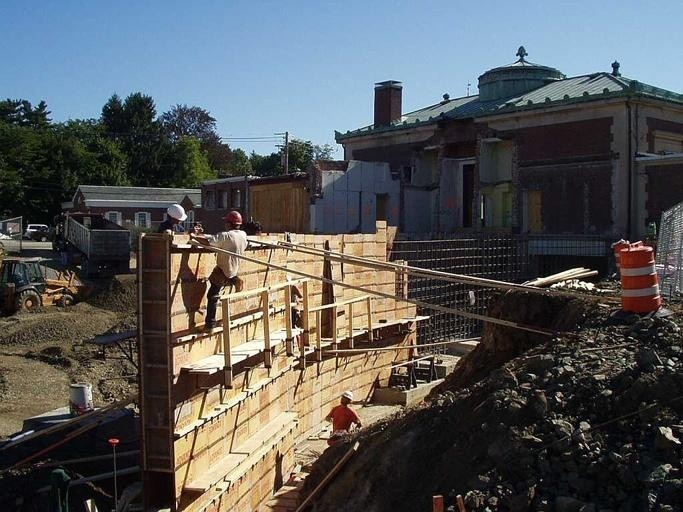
22,223,49,241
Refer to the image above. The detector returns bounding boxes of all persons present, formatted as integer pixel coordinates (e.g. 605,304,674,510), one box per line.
155,203,203,234
189,211,247,332
324,391,362,442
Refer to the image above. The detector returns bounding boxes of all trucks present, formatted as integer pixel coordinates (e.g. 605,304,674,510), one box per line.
51,211,130,276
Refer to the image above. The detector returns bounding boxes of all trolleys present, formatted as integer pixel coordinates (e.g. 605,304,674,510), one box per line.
327,419,357,446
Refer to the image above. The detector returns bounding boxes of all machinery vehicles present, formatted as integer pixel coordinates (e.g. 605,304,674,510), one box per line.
0,256,91,314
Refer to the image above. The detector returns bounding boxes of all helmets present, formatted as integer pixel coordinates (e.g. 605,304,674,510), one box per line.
222,211,243,225
340,391,353,403
167,204,188,221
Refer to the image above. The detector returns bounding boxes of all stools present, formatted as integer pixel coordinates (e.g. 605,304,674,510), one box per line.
388,362,418,391
413,356,439,383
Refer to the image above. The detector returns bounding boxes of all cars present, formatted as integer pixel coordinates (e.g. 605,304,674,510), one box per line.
30,228,55,243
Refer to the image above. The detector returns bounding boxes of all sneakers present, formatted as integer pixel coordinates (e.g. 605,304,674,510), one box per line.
199,326,212,333
234,278,243,292
